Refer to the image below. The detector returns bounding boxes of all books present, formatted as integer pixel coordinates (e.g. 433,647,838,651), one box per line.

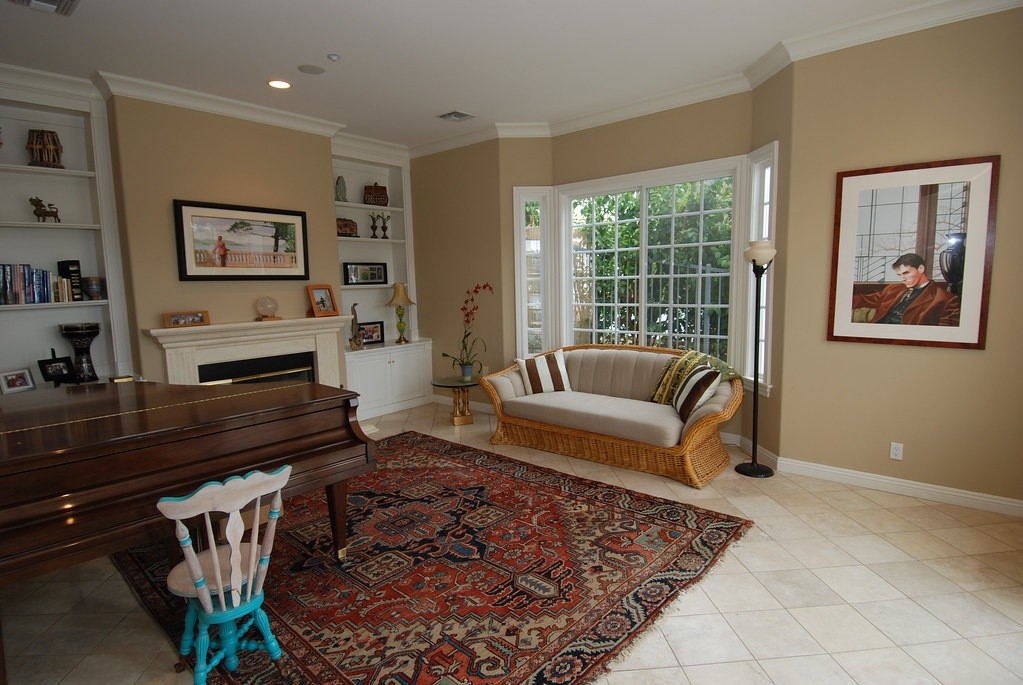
0,260,84,306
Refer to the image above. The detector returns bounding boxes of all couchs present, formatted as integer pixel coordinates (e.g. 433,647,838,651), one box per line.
479,345,743,491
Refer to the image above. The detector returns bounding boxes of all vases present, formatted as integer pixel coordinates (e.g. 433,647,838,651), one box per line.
939,233,966,295
460,365,472,381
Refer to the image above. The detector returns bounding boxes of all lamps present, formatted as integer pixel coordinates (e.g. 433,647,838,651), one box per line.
733,241,777,478
384,282,415,344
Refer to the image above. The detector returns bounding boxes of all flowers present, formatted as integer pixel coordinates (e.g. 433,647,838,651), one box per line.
440,283,496,372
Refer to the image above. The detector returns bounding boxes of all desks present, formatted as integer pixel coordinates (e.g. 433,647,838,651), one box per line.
431,376,482,426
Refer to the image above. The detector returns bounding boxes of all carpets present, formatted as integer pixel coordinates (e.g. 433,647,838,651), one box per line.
109,430,755,685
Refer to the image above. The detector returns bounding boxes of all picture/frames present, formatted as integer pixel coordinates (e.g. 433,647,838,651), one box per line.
163,310,209,328
826,154,1001,349
307,285,339,317
174,198,310,281
358,321,384,345
0,368,36,395
343,261,388,285
37,356,76,382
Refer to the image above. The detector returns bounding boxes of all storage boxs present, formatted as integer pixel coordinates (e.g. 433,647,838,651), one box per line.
213,502,284,541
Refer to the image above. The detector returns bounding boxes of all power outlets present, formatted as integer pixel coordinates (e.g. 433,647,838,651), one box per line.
889,442,903,460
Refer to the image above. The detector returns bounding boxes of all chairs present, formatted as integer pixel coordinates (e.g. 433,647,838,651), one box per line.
156,464,294,685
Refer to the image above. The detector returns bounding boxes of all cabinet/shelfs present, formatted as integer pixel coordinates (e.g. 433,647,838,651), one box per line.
335,201,406,286
0,163,108,309
344,342,433,422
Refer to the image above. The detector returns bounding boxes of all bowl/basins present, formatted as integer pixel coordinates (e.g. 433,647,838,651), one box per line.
82,277,105,299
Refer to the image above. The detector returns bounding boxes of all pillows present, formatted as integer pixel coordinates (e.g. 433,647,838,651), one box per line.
672,360,721,423
513,348,572,395
